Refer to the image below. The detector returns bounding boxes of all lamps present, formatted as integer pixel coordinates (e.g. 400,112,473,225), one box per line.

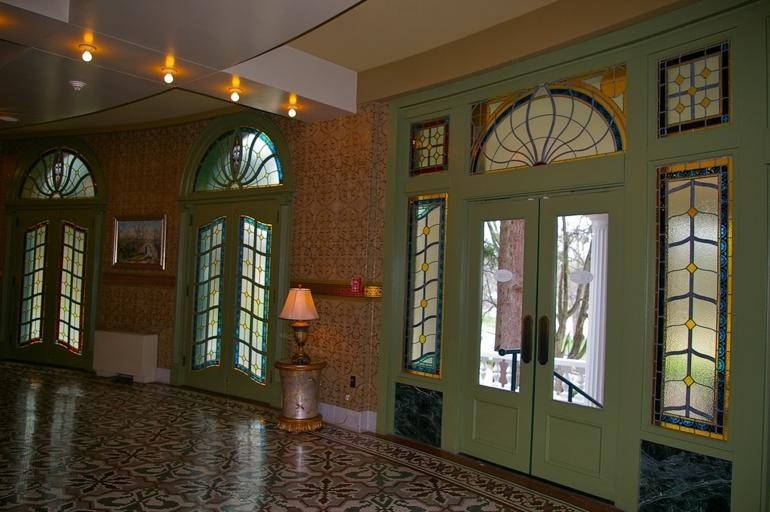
278,288,320,365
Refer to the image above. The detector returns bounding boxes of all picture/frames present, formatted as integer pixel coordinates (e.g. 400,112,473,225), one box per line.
112,213,167,271
